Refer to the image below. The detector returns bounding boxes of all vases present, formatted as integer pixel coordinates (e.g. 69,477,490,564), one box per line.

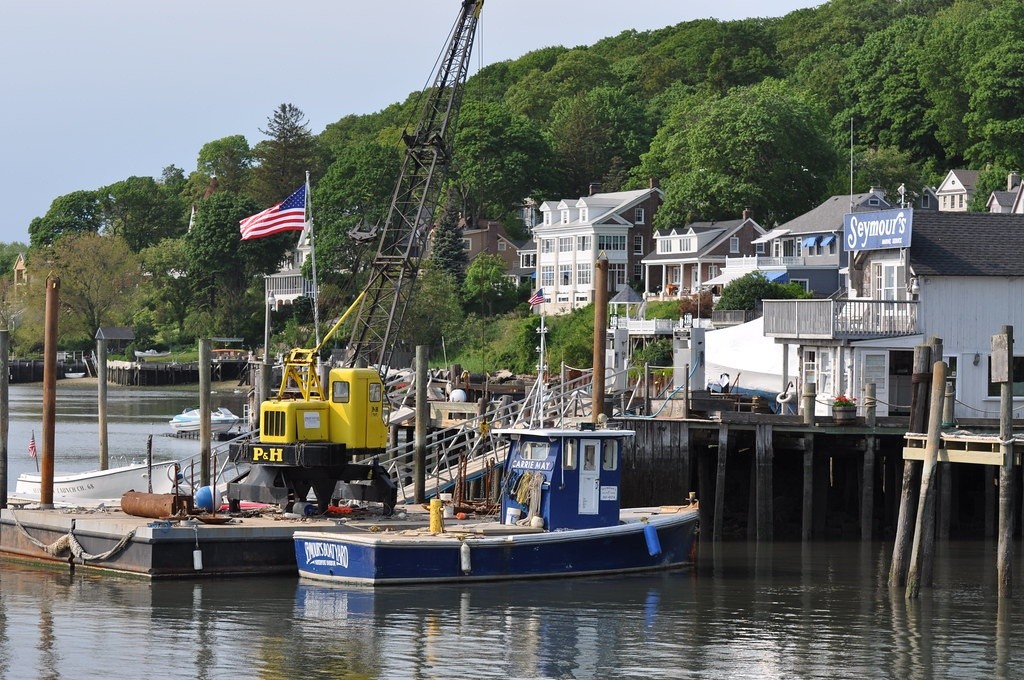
833,406,856,426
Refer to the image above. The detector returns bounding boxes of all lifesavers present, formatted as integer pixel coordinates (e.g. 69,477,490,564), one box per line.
776,389,795,405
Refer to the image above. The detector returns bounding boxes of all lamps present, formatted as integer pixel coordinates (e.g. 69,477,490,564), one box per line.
974,353,980,365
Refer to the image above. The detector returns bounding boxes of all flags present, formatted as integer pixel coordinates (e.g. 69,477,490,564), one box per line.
528,287,545,311
27,431,36,458
239,182,309,241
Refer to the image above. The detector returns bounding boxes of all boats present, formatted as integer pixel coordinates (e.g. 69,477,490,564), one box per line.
134,351,172,358
292,287,702,586
65,372,85,378
169,406,239,435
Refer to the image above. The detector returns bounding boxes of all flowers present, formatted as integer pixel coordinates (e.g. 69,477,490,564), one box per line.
832,391,857,407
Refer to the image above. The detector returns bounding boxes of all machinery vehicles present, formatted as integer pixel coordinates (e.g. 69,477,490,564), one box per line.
226,0,485,513
